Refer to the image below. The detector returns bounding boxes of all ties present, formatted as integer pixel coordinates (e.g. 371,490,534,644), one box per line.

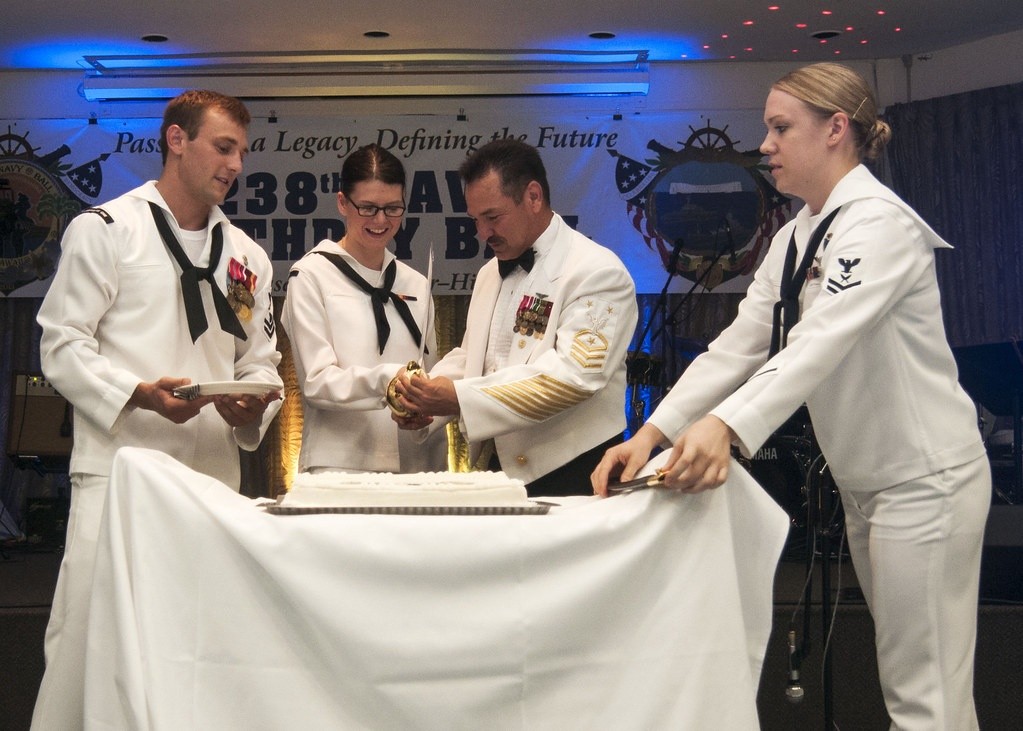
768,207,842,363
320,251,431,358
149,201,248,345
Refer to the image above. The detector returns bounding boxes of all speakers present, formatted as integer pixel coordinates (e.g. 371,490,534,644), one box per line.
756,602,1023,731
5,370,75,471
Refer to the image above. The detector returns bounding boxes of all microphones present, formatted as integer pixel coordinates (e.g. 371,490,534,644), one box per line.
60,401,72,438
723,220,738,266
786,628,805,704
666,239,682,274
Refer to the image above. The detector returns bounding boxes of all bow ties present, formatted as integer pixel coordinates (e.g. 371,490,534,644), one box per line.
498,246,538,280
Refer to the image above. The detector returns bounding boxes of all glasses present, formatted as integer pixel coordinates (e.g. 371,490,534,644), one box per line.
345,193,407,217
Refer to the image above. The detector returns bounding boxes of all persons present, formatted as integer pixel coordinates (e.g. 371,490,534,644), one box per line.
281,144,440,477
592,63,992,731
391,138,639,499
28,89,288,731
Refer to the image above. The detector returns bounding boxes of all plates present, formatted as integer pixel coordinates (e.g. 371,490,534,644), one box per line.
173,381,283,401
